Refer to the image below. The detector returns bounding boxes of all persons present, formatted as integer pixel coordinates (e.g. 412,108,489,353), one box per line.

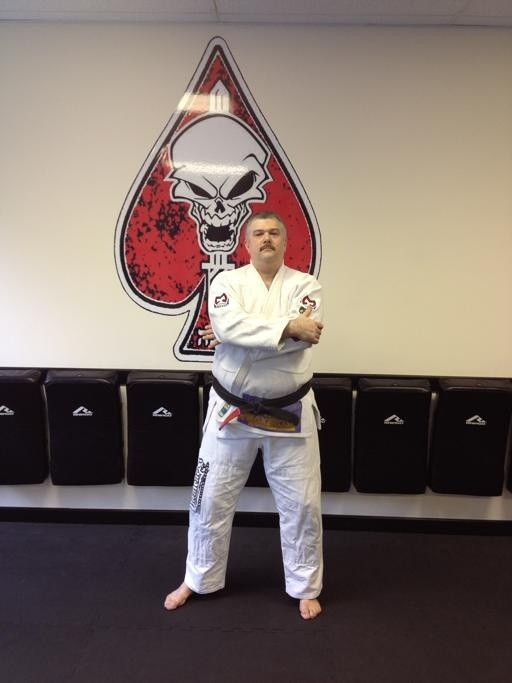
163,211,323,620
